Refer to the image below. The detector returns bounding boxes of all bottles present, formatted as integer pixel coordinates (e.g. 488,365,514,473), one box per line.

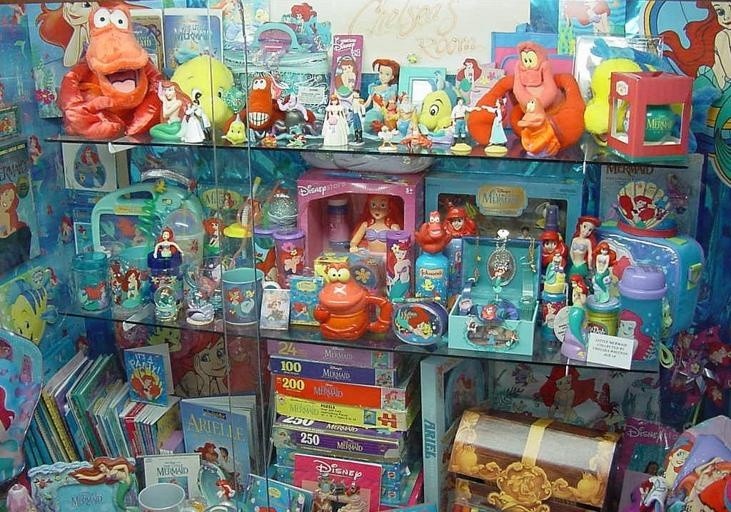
387,232,414,302
543,214,623,343
255,224,280,282
313,261,395,342
224,222,253,268
146,249,186,306
413,211,452,309
443,202,477,302
273,227,306,284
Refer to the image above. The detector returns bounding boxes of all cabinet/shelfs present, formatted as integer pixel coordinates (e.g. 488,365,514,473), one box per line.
47,125,693,381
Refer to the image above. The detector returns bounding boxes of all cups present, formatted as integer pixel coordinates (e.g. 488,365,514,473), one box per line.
184,256,266,328
619,263,669,362
70,246,150,313
137,483,186,512
